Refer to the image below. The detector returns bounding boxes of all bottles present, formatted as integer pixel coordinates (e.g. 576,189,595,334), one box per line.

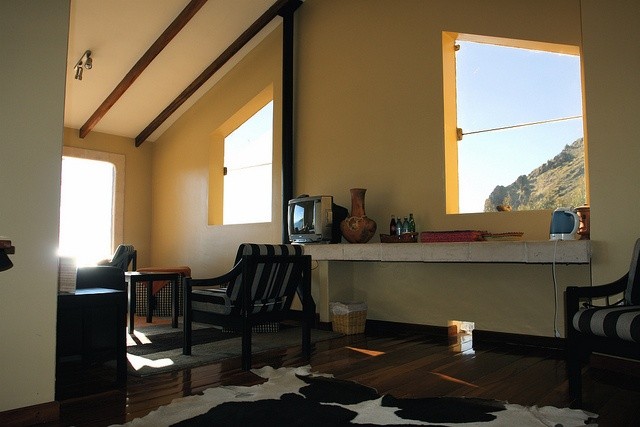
390,215,396,237
408,212,415,233
396,216,403,236
402,217,408,235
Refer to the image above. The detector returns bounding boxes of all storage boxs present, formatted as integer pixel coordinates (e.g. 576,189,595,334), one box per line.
137,267,189,317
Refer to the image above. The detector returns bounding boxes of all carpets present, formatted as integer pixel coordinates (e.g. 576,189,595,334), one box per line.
106,365,600,427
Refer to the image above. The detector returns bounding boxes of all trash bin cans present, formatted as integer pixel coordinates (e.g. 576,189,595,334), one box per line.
330,301,368,335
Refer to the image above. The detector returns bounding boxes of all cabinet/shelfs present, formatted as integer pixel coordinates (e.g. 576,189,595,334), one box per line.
55,287,128,402
298,242,594,323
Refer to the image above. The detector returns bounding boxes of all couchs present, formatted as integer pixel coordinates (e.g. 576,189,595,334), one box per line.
111,244,136,271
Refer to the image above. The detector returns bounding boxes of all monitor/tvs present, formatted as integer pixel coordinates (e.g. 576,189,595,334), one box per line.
288,196,348,244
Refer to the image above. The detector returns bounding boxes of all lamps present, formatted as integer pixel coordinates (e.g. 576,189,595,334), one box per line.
83,51,91,69
74,61,83,80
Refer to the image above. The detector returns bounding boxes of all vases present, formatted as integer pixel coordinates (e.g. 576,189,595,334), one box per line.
339,188,377,244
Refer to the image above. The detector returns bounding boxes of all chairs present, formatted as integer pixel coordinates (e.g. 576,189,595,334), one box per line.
183,244,316,371
76,266,124,290
563,239,640,409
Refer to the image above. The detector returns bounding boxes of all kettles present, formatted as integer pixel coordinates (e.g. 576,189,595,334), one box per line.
548,206,580,241
